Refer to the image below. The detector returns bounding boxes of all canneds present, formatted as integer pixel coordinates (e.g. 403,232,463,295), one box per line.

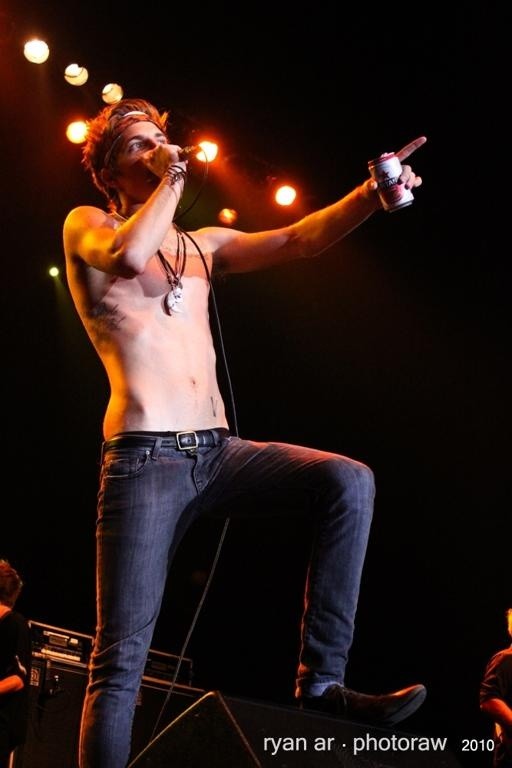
367,151,415,212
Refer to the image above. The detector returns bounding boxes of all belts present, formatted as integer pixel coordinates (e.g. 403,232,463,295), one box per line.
102,429,232,451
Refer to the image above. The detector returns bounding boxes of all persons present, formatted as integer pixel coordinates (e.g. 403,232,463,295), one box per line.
58,95,429,766
479,606,512,768
1,558,35,768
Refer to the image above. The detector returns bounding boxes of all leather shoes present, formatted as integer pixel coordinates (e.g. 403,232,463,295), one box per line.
300,685,428,727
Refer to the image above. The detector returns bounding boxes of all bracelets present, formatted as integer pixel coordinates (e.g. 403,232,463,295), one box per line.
159,165,188,206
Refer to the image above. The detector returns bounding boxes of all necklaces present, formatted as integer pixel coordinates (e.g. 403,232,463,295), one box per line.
110,206,190,318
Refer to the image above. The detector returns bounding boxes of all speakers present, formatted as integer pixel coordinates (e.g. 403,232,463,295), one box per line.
124,688,463,768
6,657,206,768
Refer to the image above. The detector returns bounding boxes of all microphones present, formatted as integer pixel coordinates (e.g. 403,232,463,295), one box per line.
144,143,201,184
